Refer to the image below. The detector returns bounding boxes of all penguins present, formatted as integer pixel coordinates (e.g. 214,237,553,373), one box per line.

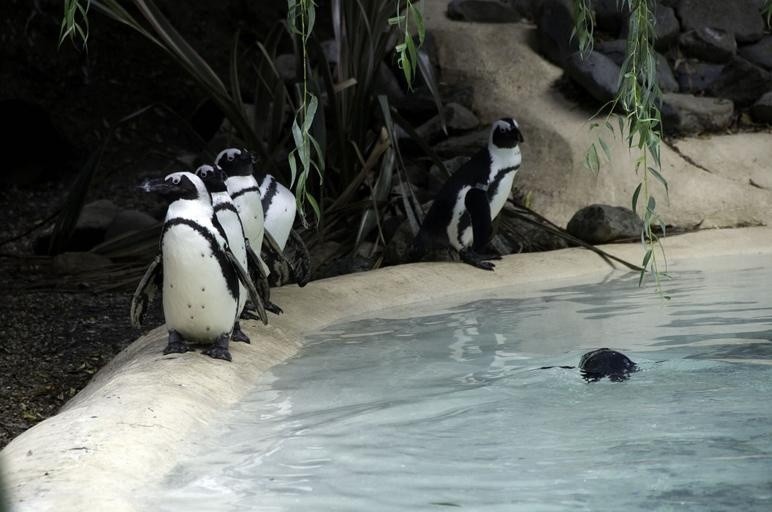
540,346,640,385
128,147,313,362
403,117,526,272
